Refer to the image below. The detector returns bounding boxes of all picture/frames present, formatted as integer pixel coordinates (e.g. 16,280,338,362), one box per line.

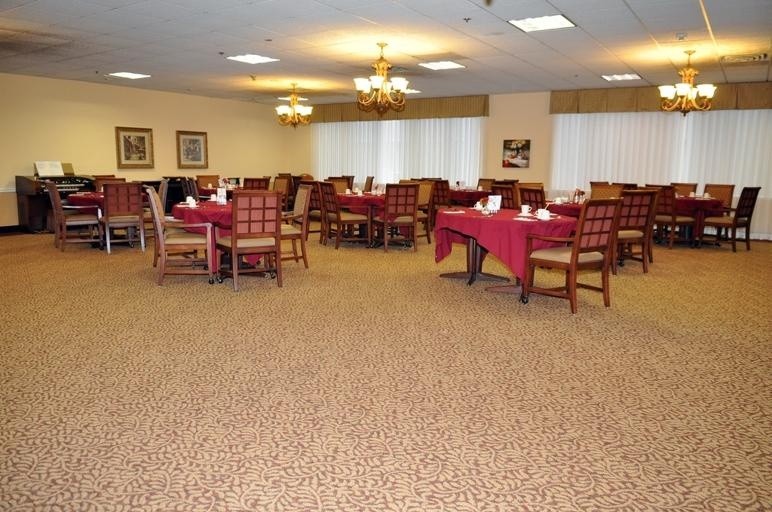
114,125,155,170
176,130,209,169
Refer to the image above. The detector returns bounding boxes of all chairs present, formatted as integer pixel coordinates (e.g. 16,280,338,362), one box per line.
45,172,762,313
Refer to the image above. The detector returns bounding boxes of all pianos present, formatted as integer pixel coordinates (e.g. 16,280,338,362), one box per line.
15,175,96,231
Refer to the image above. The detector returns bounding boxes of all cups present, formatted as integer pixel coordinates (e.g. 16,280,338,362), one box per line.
451,186,483,193
207,183,235,191
186,195,197,209
674,191,717,201
345,188,385,197
521,205,532,215
211,194,217,200
534,208,559,222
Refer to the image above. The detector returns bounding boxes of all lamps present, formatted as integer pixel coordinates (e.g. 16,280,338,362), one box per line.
658,50,716,117
352,44,422,114
276,83,313,128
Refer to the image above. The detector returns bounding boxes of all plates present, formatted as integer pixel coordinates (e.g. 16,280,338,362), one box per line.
442,211,465,215
207,200,218,202
516,213,534,217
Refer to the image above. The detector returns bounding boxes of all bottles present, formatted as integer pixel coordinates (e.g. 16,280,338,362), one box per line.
473,199,498,216
552,188,586,205
